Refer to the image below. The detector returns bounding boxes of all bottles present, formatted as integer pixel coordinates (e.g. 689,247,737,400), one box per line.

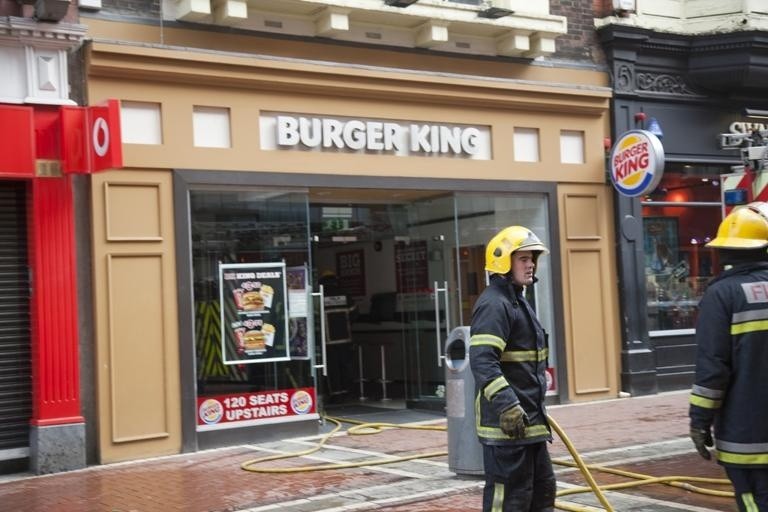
232,289,243,310
233,329,244,349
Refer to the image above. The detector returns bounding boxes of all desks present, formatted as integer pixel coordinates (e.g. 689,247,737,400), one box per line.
645,299,707,333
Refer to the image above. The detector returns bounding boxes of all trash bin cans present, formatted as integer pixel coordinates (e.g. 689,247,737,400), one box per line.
445,325,487,480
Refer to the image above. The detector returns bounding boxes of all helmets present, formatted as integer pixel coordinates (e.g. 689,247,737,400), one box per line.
483,223,551,276
702,200,768,249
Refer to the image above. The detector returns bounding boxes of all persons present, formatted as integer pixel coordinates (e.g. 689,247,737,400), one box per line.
688,204,767,512
318,269,356,395
469,223,556,511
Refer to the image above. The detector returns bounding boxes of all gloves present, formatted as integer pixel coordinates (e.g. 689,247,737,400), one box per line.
497,405,531,442
688,425,715,462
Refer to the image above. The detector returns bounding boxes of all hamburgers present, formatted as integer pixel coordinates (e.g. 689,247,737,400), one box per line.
243,291,265,311
243,329,266,350
